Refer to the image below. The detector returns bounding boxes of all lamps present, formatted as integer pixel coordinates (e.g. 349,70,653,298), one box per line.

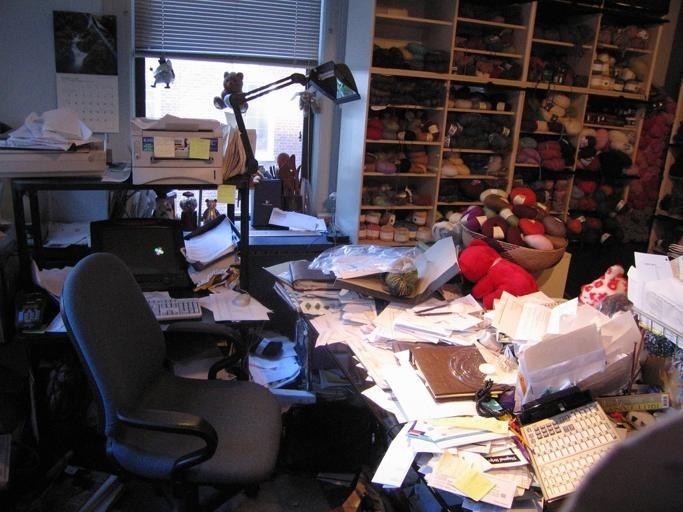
207,61,363,380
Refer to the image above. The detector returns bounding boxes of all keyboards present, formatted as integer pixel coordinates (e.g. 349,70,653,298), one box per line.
146,297,202,320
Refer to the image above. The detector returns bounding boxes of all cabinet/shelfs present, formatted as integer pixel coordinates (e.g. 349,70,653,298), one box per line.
643,82,682,259
10,172,251,484
331,0,671,242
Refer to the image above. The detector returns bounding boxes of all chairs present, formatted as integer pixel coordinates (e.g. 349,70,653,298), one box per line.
56,250,282,510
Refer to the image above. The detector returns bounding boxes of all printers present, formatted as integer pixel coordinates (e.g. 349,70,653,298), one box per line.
131,118,224,186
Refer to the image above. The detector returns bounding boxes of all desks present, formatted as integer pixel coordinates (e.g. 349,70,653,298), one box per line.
270,258,681,510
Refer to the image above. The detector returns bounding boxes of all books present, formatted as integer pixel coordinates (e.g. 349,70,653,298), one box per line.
273,280,344,307
409,344,514,399
289,261,342,290
334,238,459,302
180,217,239,294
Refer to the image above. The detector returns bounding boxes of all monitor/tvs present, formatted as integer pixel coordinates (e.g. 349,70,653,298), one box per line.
91,217,184,277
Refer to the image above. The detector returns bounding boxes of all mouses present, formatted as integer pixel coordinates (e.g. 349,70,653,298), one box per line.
232,292,252,308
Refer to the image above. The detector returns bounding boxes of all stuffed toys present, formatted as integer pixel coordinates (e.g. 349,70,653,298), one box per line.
459,238,539,310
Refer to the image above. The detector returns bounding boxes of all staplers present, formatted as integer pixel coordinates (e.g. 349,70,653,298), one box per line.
521,386,594,423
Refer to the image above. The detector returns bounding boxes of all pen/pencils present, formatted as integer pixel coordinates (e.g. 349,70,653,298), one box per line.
416,304,453,315
256,164,277,181
508,421,535,461
277,226,308,231
